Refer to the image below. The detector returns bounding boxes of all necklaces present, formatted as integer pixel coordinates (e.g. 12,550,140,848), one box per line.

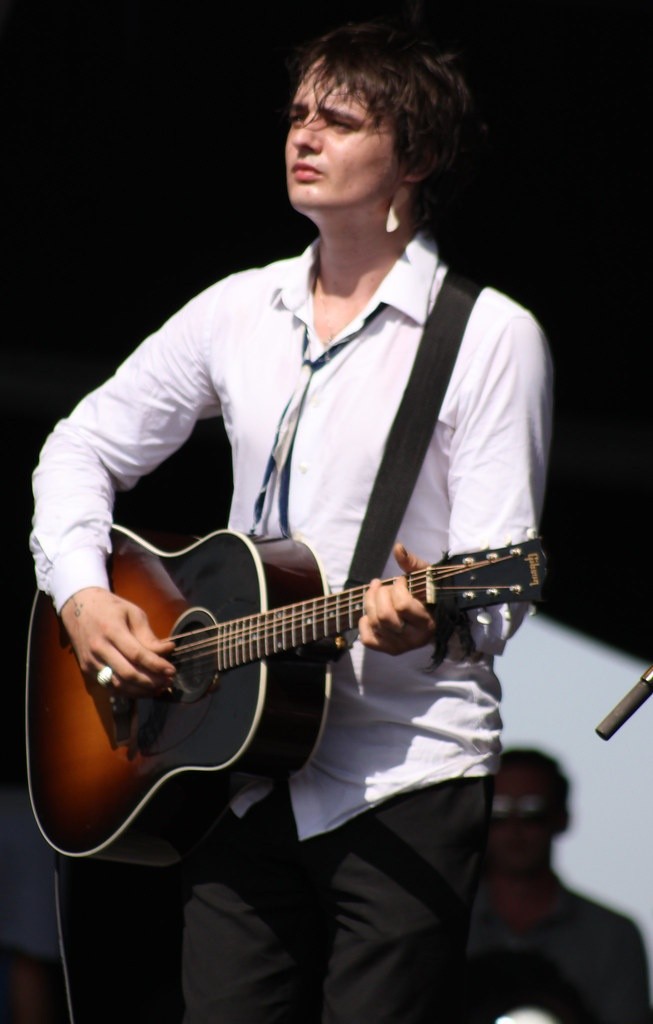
316,273,351,359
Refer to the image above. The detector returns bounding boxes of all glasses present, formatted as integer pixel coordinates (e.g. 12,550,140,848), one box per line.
492,797,558,822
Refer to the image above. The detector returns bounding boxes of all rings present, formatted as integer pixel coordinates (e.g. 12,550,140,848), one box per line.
96,665,112,685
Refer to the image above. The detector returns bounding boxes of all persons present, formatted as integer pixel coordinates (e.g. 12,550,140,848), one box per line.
467,751,652,1024
29,23,553,1024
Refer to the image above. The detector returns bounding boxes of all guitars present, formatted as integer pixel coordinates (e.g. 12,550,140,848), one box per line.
23,520,548,869
